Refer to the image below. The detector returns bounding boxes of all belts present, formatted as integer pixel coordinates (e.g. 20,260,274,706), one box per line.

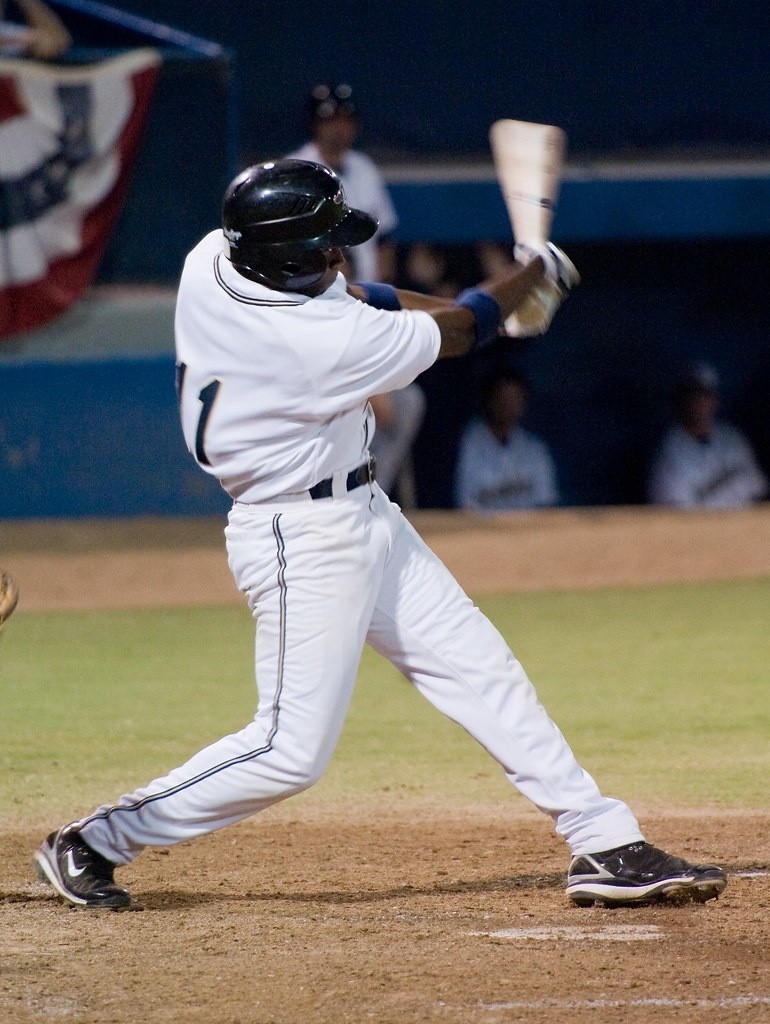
309,453,377,500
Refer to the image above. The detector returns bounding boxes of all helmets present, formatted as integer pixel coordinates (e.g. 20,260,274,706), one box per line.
220,158,381,292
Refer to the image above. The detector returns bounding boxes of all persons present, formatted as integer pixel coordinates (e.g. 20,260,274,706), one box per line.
281,89,396,289
458,379,556,509
648,355,760,511
33,154,725,909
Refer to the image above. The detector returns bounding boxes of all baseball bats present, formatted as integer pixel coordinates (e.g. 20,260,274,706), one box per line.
490,112,564,266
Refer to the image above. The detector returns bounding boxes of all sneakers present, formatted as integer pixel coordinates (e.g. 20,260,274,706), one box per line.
566,842,729,908
32,821,130,908
